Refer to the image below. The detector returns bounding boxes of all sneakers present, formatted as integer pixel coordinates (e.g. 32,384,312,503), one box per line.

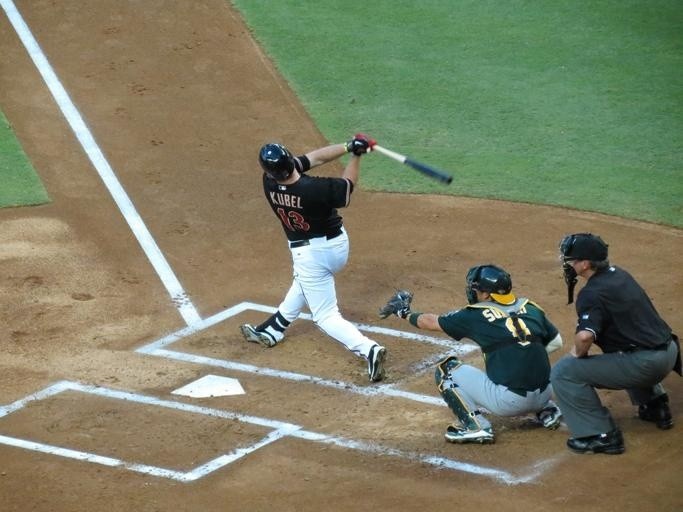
239,324,277,348
368,345,388,383
444,400,673,455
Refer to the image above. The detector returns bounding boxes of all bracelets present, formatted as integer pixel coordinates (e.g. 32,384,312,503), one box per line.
407,313,423,330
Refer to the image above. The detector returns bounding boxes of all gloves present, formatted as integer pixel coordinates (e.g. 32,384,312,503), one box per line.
345,134,376,156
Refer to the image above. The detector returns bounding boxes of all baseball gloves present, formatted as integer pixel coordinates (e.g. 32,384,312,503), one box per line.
380,290,413,319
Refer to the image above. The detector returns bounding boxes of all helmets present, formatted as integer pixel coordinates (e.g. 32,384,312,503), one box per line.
466,233,607,305
259,143,294,181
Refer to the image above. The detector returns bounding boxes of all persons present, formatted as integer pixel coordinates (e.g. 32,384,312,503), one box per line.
551,230,676,454
380,265,565,444
238,136,389,384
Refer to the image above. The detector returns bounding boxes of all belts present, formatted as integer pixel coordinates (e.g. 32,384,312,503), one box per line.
291,229,343,248
496,381,548,397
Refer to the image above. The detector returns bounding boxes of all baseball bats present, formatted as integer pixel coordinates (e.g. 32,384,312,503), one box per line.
370,144,452,185
